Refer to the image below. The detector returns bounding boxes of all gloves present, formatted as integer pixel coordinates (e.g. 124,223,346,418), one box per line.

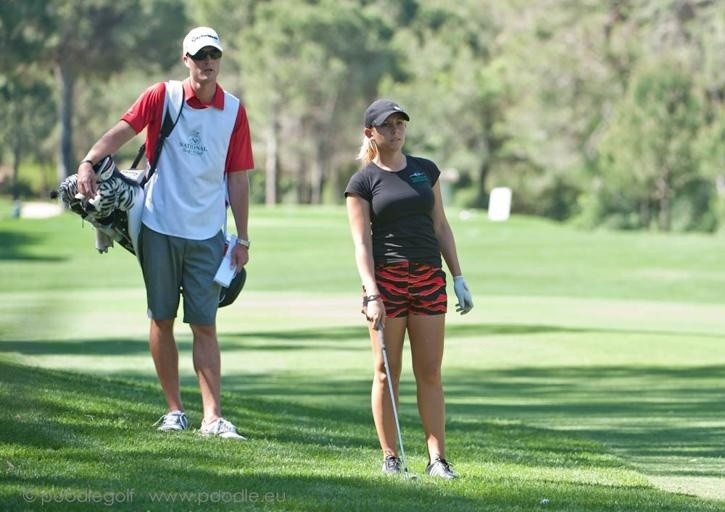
453,276,473,314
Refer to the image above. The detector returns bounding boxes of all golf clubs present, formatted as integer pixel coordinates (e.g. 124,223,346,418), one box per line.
377,322,416,481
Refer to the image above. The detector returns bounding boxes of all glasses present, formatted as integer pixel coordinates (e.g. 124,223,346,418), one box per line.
187,49,222,60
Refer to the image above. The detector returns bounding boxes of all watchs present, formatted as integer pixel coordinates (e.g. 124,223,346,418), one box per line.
235,237,252,248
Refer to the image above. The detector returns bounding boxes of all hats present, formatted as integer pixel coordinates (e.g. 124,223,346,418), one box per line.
182,27,223,55
365,98,410,128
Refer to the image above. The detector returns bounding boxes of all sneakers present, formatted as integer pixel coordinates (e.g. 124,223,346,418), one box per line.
198,416,247,441
153,410,188,432
425,456,460,480
381,455,416,480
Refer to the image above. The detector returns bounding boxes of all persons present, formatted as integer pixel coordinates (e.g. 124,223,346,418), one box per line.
77,26,254,441
343,99,474,480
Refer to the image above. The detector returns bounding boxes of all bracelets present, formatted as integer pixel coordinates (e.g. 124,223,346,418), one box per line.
77,159,94,164
363,293,382,301
